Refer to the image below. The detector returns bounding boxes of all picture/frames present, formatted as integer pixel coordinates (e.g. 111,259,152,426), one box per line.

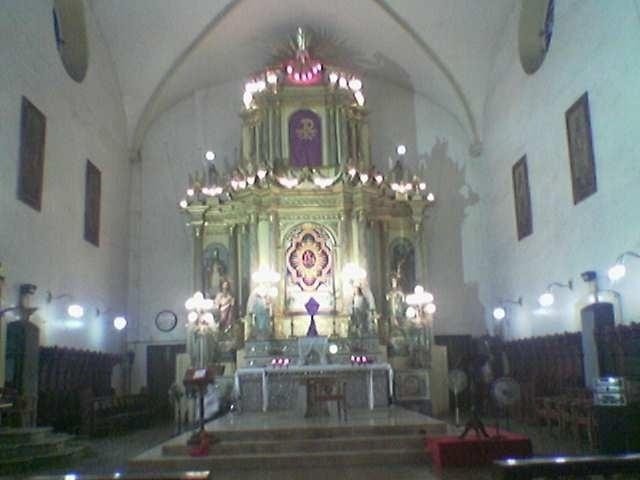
85,160,101,248
512,154,534,240
565,91,596,205
16,95,46,212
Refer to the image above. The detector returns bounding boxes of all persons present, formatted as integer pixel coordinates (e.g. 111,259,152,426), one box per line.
215,281,235,339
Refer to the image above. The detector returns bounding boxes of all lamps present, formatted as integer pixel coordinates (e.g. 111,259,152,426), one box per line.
47,291,83,318
493,251,640,319
97,308,127,330
